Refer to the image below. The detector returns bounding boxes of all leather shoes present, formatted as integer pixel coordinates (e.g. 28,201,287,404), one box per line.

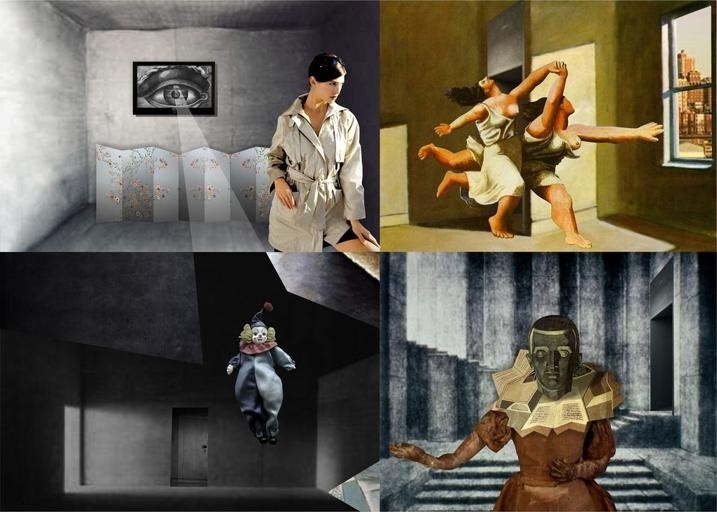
259,437,277,445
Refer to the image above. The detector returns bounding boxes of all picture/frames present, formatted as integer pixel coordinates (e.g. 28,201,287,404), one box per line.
130,60,217,117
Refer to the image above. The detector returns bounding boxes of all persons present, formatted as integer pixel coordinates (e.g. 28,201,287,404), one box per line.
416,97,668,250
386,312,623,510
225,300,297,447
435,57,560,239
264,51,378,251
